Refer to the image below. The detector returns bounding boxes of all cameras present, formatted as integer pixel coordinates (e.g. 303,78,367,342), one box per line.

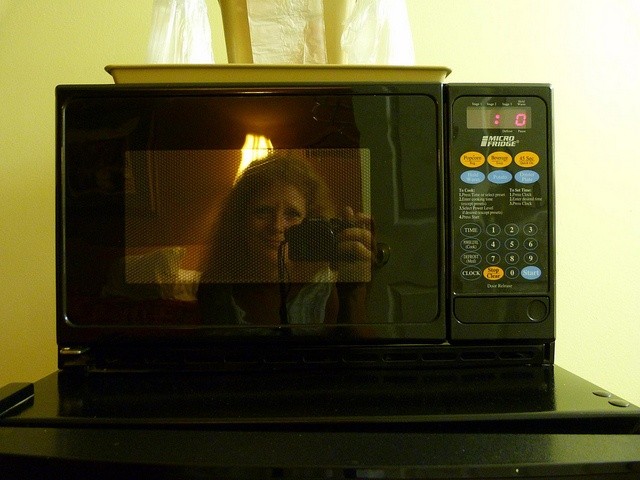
280,215,367,264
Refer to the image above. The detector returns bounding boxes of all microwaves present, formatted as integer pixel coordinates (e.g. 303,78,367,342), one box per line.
54,81,558,382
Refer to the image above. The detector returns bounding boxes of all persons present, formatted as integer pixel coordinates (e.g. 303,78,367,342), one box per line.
192,153,381,340
